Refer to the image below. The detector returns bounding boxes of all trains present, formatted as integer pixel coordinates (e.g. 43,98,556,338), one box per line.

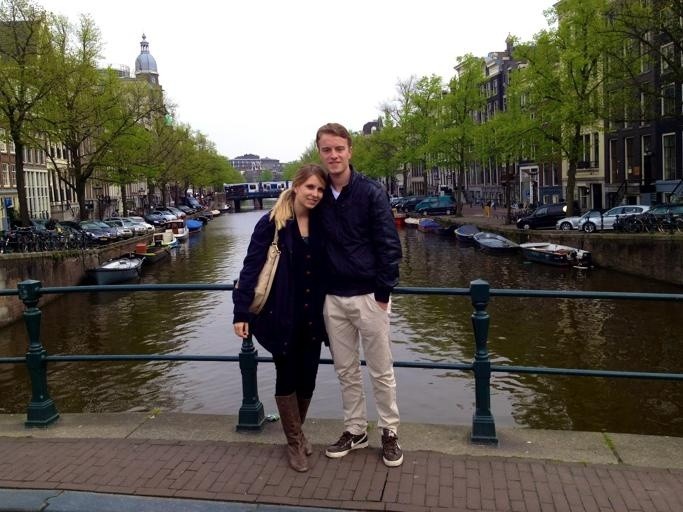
223,180,293,194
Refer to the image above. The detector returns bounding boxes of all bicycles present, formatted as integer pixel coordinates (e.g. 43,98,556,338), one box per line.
1,231,94,253
614,208,683,235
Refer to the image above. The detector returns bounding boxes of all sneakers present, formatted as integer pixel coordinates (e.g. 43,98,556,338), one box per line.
381,430,402,467
325,432,367,458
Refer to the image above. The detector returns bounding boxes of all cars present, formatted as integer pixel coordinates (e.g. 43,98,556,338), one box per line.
647,207,683,218
387,194,458,216
578,205,651,232
555,208,611,230
8,193,203,244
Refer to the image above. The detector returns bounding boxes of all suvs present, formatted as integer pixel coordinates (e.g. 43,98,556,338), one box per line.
516,203,580,230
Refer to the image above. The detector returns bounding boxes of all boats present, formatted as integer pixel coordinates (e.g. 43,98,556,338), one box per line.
394,216,591,263
512,256,587,278
146,253,172,271
135,204,234,262
90,289,139,306
90,253,146,285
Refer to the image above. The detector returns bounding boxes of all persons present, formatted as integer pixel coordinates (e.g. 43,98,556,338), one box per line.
310,121,404,467
231,164,331,472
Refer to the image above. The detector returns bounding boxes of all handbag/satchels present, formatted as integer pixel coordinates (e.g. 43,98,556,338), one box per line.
234,245,281,315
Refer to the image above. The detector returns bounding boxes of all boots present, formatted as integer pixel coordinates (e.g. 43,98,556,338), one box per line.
274,390,312,472
